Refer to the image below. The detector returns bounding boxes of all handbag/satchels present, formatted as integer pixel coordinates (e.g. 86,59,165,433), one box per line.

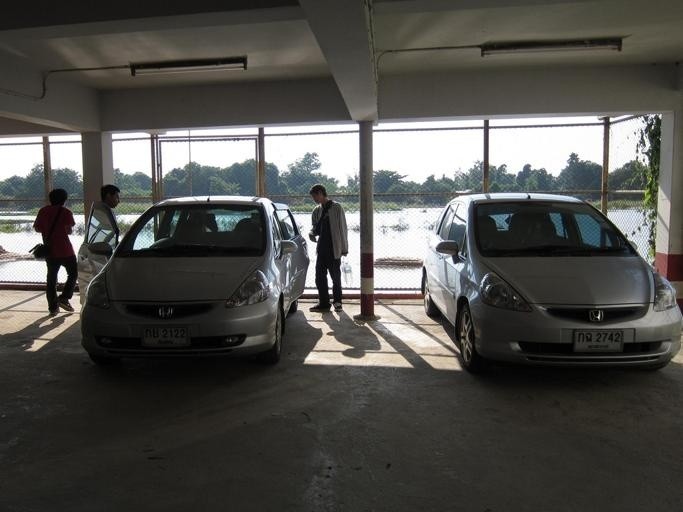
29,242,48,261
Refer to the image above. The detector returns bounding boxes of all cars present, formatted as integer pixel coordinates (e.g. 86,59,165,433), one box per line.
71,196,308,371
419,193,681,376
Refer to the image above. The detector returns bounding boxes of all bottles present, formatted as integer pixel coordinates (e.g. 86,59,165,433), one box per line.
343,261,353,286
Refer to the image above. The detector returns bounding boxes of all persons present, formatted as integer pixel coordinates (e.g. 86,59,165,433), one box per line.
32,189,77,316
101,184,121,246
308,184,349,312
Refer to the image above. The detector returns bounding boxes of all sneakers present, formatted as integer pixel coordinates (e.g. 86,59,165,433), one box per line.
310,303,330,312
56,295,74,312
50,309,60,316
334,302,342,312
